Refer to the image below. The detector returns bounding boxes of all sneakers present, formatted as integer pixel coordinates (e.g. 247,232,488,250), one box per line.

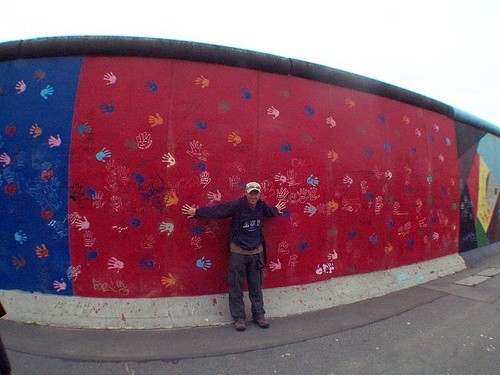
253,317,269,328
235,320,246,330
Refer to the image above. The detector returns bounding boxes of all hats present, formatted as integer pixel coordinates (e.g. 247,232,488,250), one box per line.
245,182,261,193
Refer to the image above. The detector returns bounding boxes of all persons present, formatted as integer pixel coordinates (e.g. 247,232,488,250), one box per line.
180,181,287,330
